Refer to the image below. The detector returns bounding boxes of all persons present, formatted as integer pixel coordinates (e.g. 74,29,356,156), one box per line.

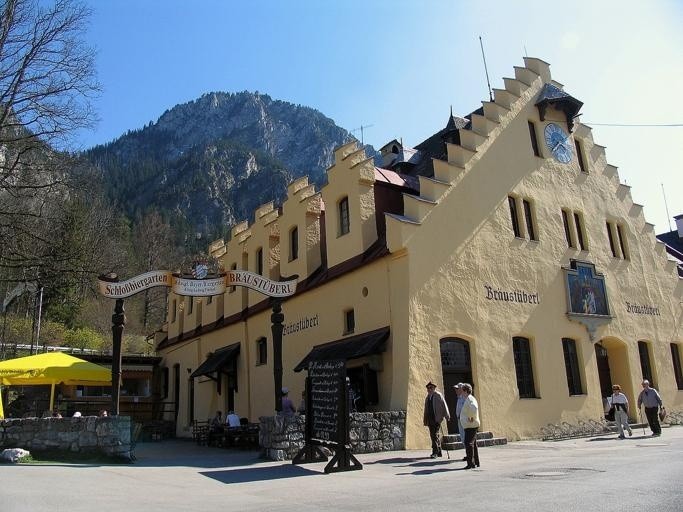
282,387,295,412
204,409,224,449
452,381,469,461
221,410,241,441
297,390,305,414
50,407,111,418
609,384,632,439
459,383,479,469
422,381,450,458
636,379,663,437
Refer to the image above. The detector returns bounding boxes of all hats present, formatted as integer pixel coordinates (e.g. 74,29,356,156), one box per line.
281,386,290,394
426,381,437,387
452,382,463,388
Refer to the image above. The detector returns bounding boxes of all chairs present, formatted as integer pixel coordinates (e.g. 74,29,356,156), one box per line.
130,423,142,462
192,418,260,452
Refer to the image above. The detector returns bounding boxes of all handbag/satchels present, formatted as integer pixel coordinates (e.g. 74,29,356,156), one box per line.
659,405,666,421
607,407,616,421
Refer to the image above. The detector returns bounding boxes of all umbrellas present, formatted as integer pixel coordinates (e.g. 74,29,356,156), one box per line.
0,350,123,415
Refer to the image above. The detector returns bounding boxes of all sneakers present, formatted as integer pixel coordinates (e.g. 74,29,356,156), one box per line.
430,454,479,469
